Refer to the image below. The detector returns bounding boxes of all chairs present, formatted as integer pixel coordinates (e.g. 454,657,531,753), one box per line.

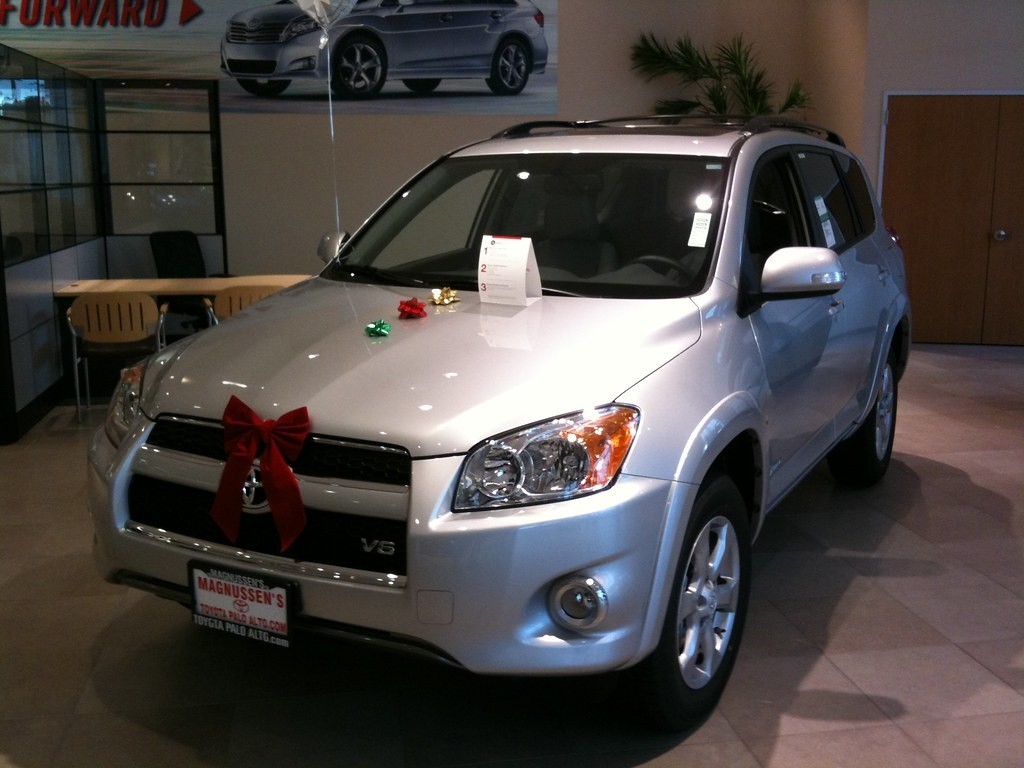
64,291,169,425
202,285,285,330
148,230,236,331
528,186,619,277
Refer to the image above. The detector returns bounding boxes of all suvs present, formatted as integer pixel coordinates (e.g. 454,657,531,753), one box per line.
219,0,549,101
87,114,914,731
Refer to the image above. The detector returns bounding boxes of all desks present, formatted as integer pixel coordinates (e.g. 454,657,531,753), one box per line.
53,276,316,295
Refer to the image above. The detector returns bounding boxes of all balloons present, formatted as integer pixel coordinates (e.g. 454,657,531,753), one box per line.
297,0,359,49
